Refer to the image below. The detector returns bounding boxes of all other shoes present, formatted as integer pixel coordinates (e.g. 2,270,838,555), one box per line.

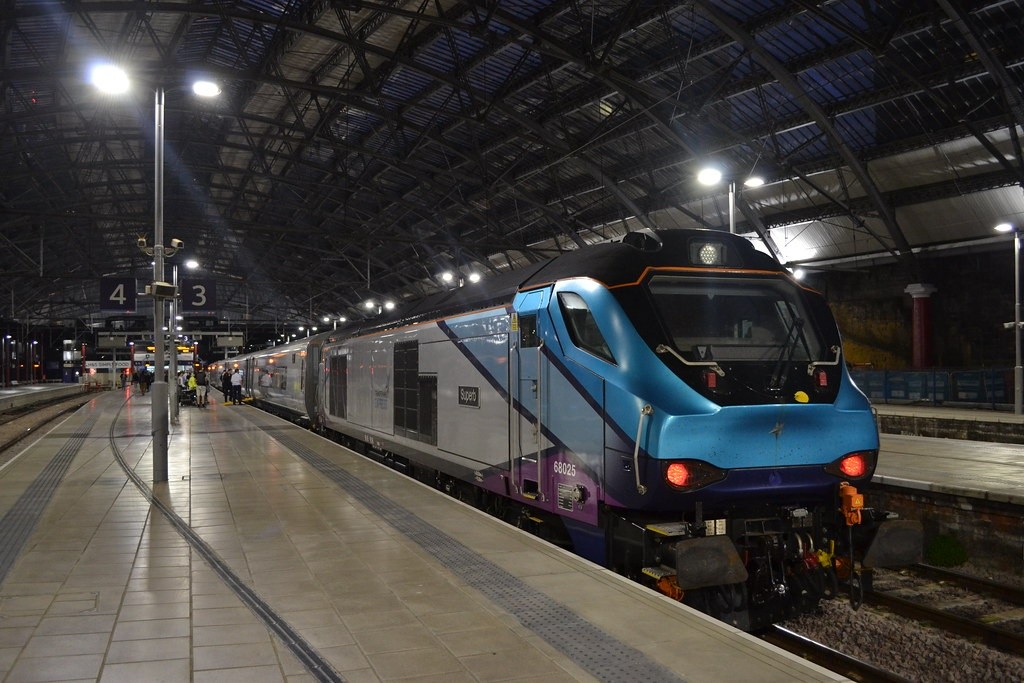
193,398,210,408
224,396,244,405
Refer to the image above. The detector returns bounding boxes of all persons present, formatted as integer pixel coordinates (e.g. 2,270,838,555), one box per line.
223,369,235,403
132,369,227,403
231,370,246,406
194,367,208,409
257,369,275,401
120,370,128,392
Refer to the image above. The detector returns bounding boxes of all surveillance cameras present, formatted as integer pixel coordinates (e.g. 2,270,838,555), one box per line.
137,238,147,248
170,239,184,249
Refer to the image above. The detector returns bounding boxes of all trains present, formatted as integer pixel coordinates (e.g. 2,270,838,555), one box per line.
207,227,881,616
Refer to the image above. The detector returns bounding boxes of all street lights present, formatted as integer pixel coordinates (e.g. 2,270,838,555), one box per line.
299,326,318,337
150,258,201,424
269,338,281,347
994,222,1024,416
442,272,480,287
282,334,297,342
87,63,222,481
698,167,765,234
366,302,394,315
324,317,347,330
